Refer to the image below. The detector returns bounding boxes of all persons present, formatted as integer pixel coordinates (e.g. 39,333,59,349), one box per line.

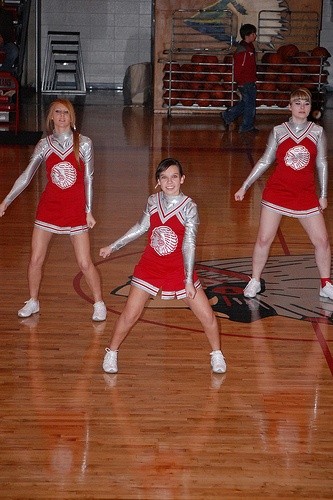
0,97,108,321
218,24,261,132
99,158,226,373
234,86,333,299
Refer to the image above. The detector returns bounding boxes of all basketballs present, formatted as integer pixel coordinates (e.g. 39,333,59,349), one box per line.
163,44,329,107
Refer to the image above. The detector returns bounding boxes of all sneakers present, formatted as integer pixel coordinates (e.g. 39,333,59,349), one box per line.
319,281,333,300
102,347,118,373
242,276,261,298
17,298,39,317
209,350,227,374
91,301,106,321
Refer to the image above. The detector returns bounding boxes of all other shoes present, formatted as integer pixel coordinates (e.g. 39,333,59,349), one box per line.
245,128,259,134
220,111,229,130
